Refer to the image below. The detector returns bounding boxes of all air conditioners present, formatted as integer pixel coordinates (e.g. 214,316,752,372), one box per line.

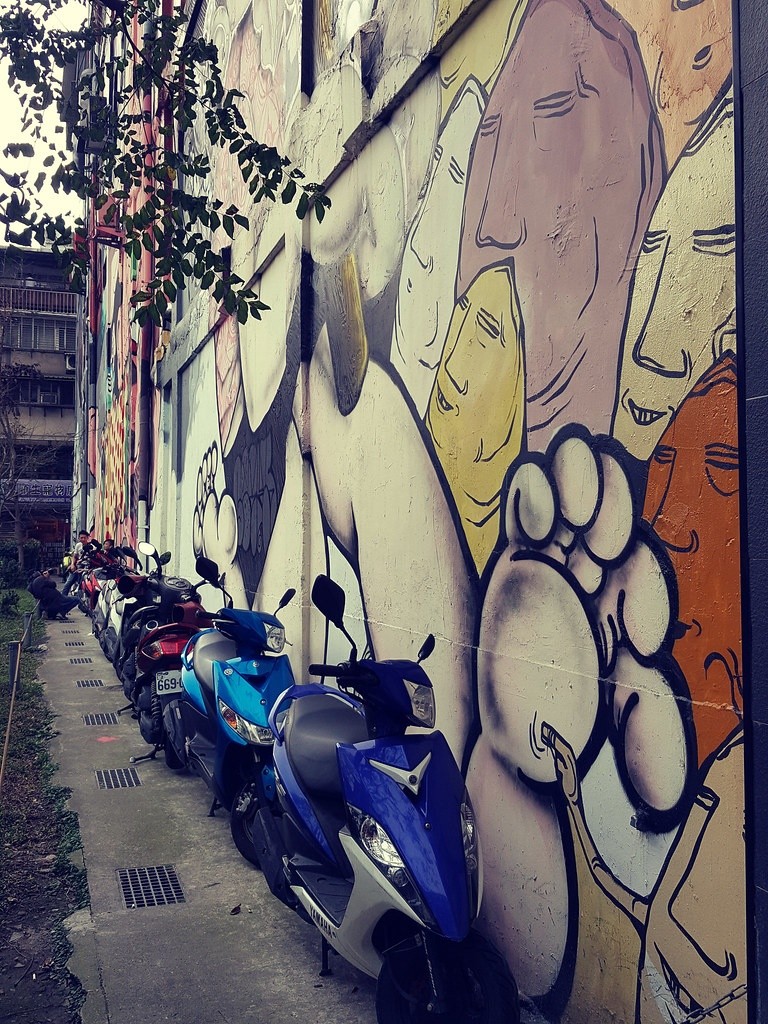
65,353,75,372
81,96,108,151
40,393,58,405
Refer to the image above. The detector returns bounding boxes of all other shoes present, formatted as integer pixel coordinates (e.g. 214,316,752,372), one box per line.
56,612,68,620
42,611,50,619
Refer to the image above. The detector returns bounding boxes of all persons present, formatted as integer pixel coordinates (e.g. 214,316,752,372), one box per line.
27,569,78,621
62,529,101,612
102,539,118,563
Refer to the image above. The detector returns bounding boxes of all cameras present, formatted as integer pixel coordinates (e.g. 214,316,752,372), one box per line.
44,568,55,575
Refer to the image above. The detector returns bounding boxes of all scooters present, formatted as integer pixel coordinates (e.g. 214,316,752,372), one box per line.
59,534,297,870
259,574,524,1024
